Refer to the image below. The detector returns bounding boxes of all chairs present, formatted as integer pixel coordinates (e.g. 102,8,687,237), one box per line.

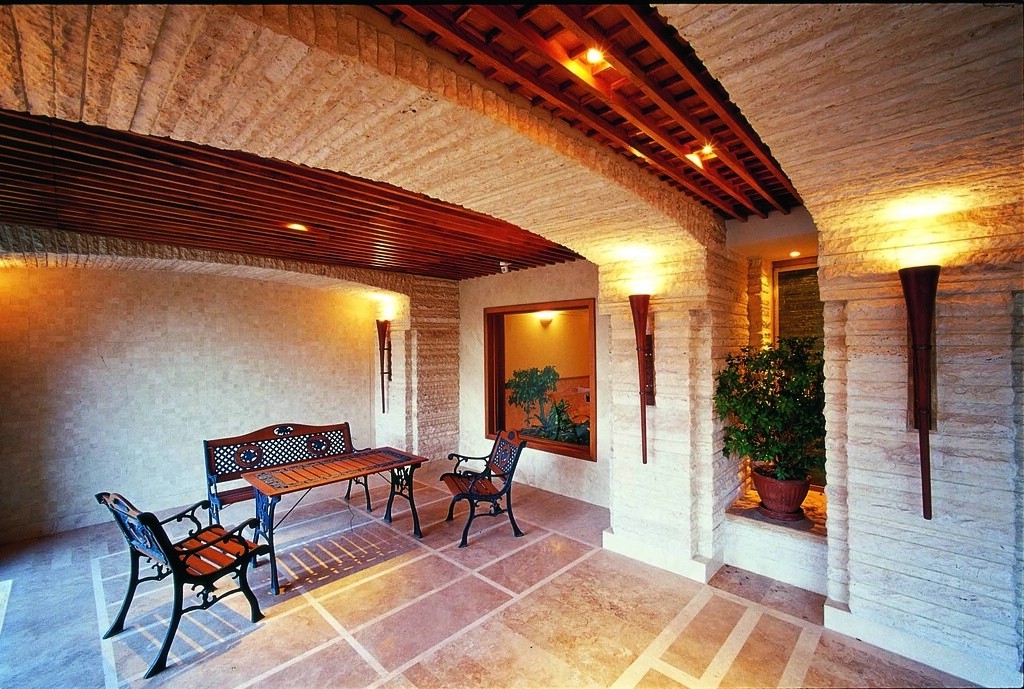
95,492,272,680
440,429,527,549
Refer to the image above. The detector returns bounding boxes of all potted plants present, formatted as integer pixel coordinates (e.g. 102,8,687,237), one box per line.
713,334,824,521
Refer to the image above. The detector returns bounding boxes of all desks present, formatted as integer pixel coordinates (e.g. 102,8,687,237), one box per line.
241,446,429,595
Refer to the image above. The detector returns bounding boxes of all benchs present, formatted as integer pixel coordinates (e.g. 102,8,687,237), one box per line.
203,422,372,514
